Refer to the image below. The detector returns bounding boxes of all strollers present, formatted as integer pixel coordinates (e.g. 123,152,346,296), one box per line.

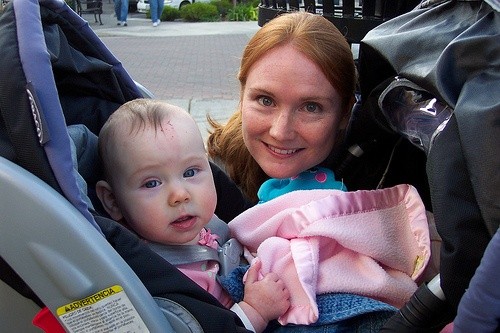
0,0,500,333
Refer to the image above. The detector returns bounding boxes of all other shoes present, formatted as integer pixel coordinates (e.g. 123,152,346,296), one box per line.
157,19,161,24
153,22,158,27
122,22,128,26
117,21,121,25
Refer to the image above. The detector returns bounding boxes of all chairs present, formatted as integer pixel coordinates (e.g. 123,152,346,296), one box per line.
75,0,104,25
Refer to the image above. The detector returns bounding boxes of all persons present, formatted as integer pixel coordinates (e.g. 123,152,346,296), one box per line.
149,0,164,27
205,11,439,282
112,0,129,26
439,226,500,333
95,98,291,333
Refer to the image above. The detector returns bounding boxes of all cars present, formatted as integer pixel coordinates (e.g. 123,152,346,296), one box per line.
136,0,211,14
